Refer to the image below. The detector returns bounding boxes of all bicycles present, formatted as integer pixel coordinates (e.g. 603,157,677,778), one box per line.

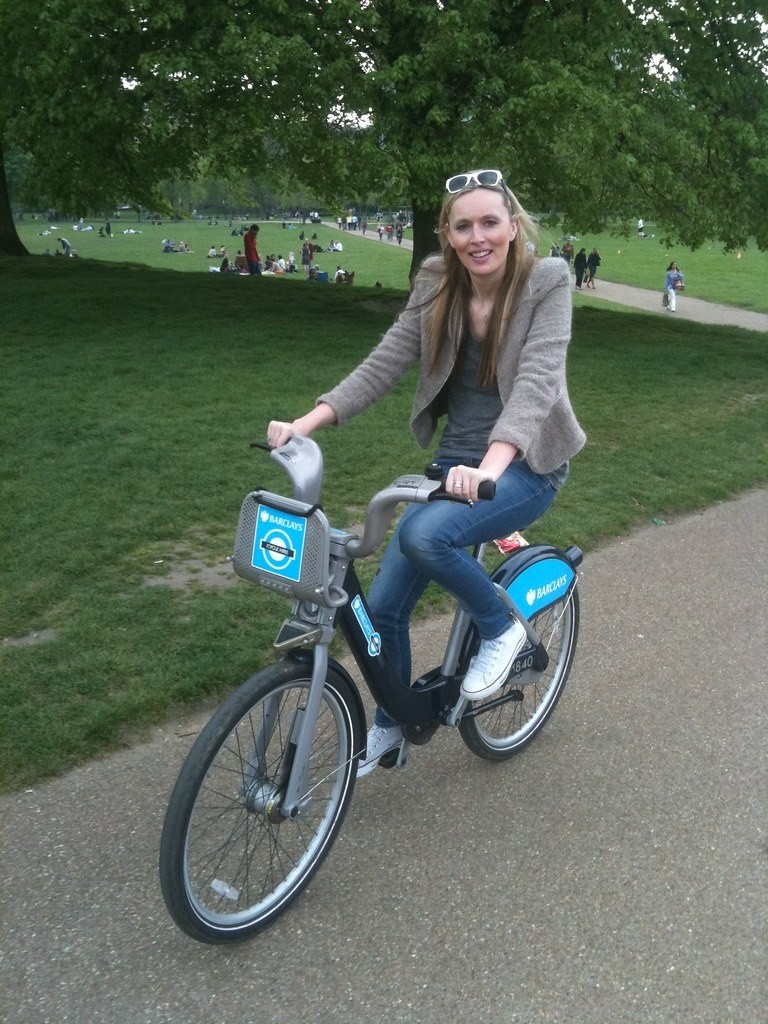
158,431,585,944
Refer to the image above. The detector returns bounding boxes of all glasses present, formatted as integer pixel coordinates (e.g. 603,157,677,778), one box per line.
446,170,502,194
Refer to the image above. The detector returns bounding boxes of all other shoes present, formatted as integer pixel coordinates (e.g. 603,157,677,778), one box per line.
591,285,595,289
574,286,578,290
671,309,675,312
586,284,590,288
667,306,670,310
579,288,583,290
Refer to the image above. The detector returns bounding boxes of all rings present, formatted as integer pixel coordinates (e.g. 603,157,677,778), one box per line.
267,437,272,440
454,481,463,487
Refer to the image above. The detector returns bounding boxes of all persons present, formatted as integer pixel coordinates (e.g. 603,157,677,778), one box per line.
638,217,644,232
665,262,685,311
163,240,194,254
278,210,414,285
239,216,247,221
35,210,143,237
561,239,574,264
573,248,587,290
45,237,78,258
563,232,580,241
361,220,367,235
230,225,250,235
147,209,232,226
265,167,588,779
549,240,561,257
586,247,601,289
637,232,655,237
208,245,296,276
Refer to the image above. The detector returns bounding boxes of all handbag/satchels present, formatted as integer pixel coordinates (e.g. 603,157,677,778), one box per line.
583,272,588,283
675,280,685,291
662,292,669,306
377,228,381,233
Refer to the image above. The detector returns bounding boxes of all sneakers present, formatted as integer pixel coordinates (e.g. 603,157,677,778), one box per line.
460,618,527,700
356,723,404,779
